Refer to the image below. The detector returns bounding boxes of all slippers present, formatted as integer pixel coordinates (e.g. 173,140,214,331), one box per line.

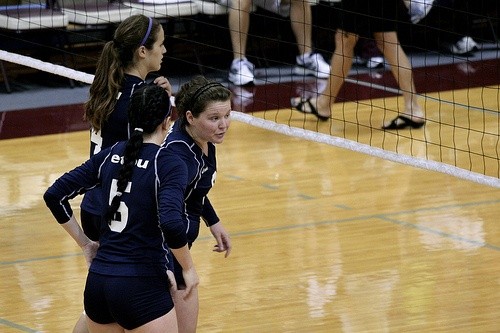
382,115,424,130
294,96,329,121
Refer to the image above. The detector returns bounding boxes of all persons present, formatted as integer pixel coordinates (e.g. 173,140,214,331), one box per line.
213,0,331,86
43,84,200,333
291,0,426,130
72,14,234,333
359,0,479,68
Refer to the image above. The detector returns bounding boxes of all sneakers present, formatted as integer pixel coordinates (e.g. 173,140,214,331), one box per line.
229,58,255,86
449,36,478,55
292,52,330,78
363,47,385,69
405,0,434,24
